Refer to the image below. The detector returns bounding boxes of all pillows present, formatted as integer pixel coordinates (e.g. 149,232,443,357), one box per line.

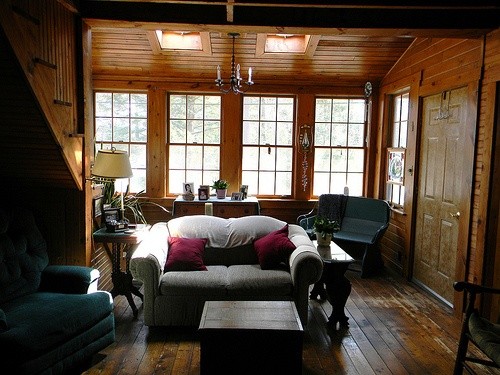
164,236,209,274
251,223,297,270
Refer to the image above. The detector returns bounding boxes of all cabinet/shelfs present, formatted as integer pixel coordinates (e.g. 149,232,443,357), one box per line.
172,194,260,220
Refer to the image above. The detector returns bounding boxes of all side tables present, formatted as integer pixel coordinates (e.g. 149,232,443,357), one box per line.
91,223,151,319
311,239,355,336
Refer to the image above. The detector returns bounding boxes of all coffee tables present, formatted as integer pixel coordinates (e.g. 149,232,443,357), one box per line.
197,301,304,375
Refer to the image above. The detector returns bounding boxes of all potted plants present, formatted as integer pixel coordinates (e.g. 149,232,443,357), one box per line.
99,177,172,229
312,215,340,247
208,178,230,199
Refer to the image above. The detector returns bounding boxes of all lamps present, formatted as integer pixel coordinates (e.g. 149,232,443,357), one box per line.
85,146,133,184
298,123,314,192
214,32,254,96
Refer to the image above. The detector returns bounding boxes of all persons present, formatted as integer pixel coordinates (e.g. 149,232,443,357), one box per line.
200,191,207,199
185,184,193,194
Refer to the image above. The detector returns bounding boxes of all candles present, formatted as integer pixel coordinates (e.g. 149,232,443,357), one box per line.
119,179,123,209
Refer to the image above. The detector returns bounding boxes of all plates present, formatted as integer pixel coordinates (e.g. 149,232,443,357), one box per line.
124,230,135,233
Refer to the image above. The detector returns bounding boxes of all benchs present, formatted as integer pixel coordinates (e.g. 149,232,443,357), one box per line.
296,193,390,279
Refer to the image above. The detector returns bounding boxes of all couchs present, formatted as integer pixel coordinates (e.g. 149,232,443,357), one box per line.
127,215,323,335
0,201,116,375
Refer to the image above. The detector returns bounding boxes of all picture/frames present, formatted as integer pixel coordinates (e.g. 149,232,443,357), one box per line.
199,185,210,199
198,188,209,201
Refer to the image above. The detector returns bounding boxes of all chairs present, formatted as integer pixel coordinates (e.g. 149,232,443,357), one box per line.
453,279,500,375
